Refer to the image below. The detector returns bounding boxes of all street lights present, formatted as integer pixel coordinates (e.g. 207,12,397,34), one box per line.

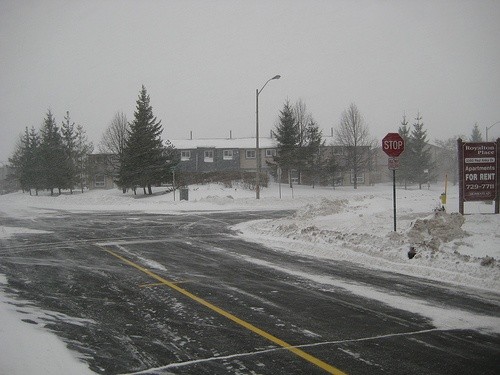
256,75,282,199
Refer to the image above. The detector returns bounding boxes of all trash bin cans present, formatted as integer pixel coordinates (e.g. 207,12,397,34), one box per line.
179,188,189,201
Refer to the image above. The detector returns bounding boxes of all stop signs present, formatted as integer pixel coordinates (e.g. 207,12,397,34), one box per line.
382,132,404,158
